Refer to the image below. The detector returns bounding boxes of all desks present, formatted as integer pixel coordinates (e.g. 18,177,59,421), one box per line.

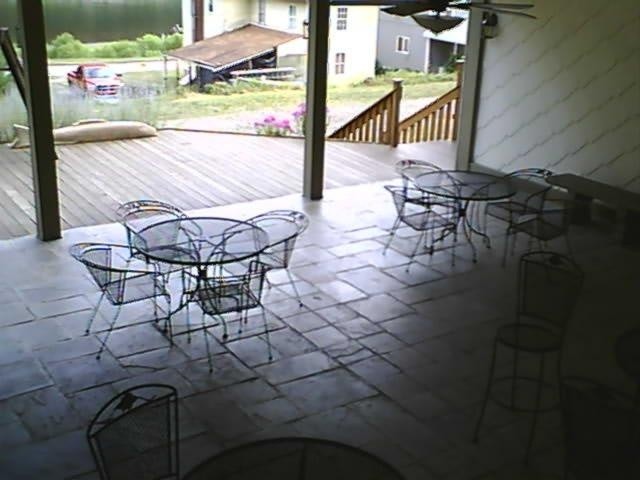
614,326,638,381
132,213,270,339
175,424,400,476
410,170,517,249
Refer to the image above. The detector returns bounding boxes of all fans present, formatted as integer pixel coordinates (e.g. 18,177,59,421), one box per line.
332,0,536,35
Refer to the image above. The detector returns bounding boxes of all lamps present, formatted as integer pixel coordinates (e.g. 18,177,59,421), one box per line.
482,13,497,39
410,11,465,35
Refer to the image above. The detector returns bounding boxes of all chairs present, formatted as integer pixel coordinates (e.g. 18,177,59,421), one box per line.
184,260,277,370
395,158,462,252
71,242,176,363
80,384,186,479
114,195,205,284
215,207,309,305
468,249,594,459
471,165,553,249
558,363,640,479
383,184,456,272
497,199,575,267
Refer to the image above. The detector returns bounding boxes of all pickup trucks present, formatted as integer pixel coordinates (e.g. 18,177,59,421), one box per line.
67,63,125,100
